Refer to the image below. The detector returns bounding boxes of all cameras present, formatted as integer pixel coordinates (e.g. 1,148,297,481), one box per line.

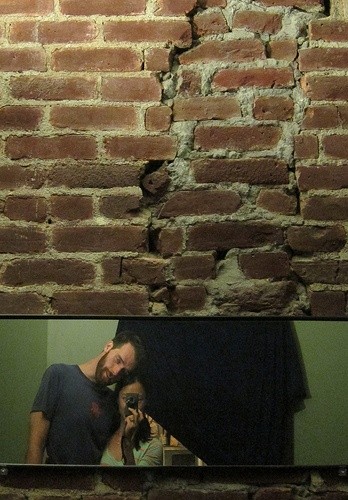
124,392,138,417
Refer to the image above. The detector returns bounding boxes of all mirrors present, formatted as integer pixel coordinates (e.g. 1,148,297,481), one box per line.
1,314,348,470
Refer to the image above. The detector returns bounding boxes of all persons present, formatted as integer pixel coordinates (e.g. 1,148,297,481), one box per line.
22,330,155,467
96,373,166,466
